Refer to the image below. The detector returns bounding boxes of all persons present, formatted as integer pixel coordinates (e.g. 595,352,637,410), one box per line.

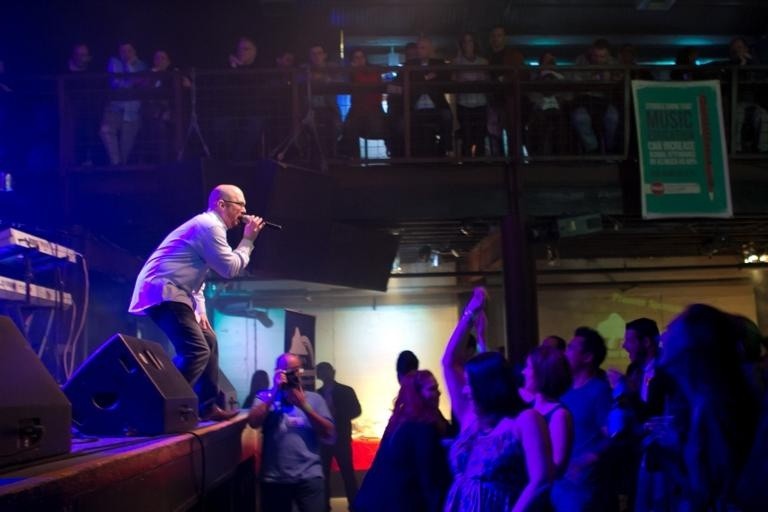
450,333,476,437
441,285,556,511
396,350,420,387
127,184,266,421
242,369,269,409
348,369,448,511
518,301,768,512
315,361,362,512
46,25,767,170
247,351,338,512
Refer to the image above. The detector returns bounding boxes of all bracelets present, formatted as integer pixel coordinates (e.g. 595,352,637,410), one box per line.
462,307,474,321
304,402,312,414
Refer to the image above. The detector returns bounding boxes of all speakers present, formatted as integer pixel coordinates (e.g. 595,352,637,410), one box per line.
62,332,199,437
0,316,72,474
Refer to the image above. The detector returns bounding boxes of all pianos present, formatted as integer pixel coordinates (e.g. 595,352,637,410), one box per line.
0,277,74,315
0,228,80,277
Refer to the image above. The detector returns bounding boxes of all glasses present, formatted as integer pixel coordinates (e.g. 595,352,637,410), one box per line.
225,201,247,207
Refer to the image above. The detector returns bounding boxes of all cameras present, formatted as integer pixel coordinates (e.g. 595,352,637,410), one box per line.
279,368,300,407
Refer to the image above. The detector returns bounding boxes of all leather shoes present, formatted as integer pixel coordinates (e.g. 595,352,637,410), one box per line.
203,406,240,421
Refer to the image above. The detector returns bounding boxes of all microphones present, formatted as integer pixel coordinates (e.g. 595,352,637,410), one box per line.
241,214,283,232
